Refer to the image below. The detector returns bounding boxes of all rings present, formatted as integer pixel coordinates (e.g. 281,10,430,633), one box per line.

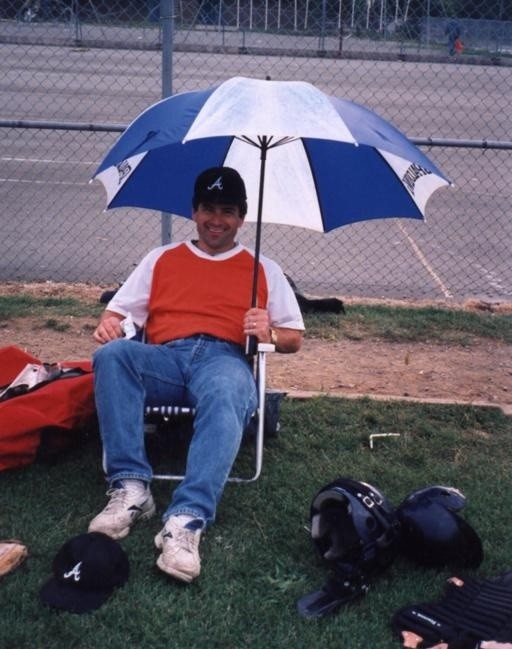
254,322,256,328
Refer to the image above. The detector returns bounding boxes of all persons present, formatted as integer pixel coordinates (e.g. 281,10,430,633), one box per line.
86,165,307,586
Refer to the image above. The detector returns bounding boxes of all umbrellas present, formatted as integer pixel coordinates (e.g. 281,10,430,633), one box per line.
85,75,455,360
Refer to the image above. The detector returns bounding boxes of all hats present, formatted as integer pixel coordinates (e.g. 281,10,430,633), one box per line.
40,532,129,614
194,167,246,204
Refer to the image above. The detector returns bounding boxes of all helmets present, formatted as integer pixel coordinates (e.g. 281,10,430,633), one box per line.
396,486,484,569
310,478,400,588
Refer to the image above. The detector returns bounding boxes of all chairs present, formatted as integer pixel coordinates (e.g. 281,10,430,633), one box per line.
101,284,275,484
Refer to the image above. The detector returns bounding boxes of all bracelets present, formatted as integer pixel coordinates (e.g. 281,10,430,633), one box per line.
270,326,277,345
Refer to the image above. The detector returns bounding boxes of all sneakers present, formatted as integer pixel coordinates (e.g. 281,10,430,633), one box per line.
0,539,27,576
88,478,156,541
153,514,207,584
